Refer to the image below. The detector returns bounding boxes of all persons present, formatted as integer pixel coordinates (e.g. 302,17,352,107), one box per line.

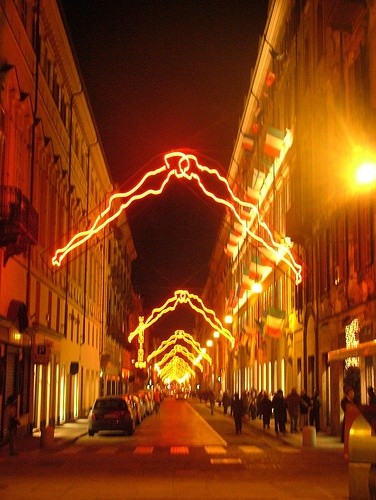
339,389,354,443
229,396,236,416
153,388,162,415
162,385,223,407
209,389,215,414
0,393,21,456
232,393,243,435
222,390,230,414
241,387,322,436
359,385,376,437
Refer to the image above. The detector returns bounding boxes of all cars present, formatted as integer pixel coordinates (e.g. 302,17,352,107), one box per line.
176,392,187,400
86,389,155,436
